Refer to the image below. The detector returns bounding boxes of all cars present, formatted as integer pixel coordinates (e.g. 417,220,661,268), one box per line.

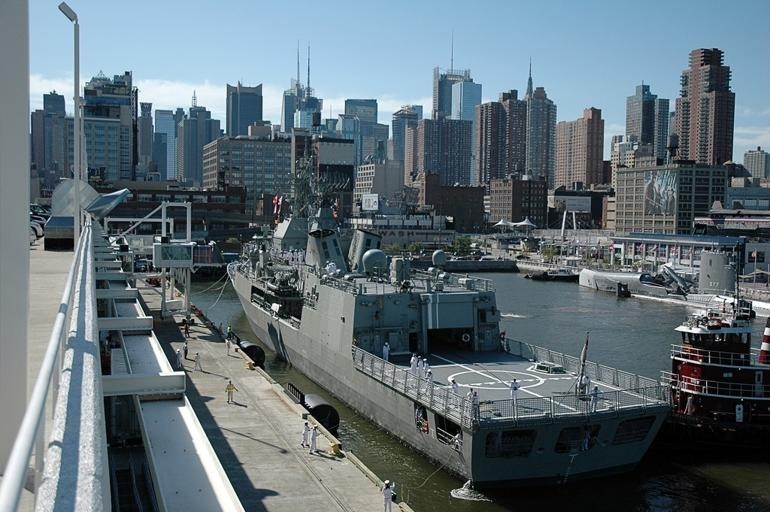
29,203,52,246
135,259,147,272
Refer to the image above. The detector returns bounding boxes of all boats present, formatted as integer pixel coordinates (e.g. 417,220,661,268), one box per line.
659,278,770,432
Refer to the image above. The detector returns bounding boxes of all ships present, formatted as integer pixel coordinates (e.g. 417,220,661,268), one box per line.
226,130,670,483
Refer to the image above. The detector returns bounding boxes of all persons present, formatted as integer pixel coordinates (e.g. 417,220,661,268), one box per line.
218,323,223,337
182,341,189,360
193,352,202,372
381,478,397,512
408,350,435,395
582,374,590,396
173,348,183,369
226,325,232,340
211,322,219,333
454,429,463,451
471,392,479,419
588,384,603,414
580,430,591,451
299,421,311,449
309,424,323,454
382,341,390,362
467,387,474,413
183,322,191,338
451,379,459,406
509,378,521,406
225,379,240,403
226,339,231,356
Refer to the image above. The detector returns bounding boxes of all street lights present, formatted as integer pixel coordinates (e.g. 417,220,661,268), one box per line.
58,2,87,252
